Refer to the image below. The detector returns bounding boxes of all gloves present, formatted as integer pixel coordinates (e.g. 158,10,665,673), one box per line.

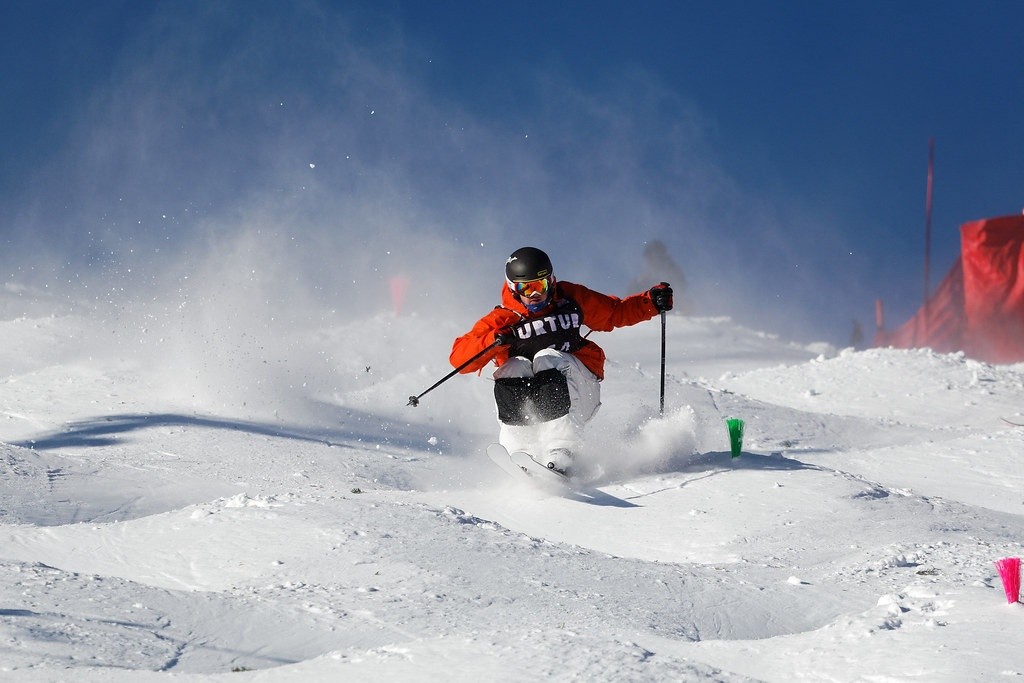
495,322,519,346
650,281,673,313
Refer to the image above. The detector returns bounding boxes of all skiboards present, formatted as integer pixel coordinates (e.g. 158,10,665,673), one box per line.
486,444,646,508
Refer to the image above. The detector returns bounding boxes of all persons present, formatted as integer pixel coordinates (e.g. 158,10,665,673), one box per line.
450,245,674,483
630,239,686,316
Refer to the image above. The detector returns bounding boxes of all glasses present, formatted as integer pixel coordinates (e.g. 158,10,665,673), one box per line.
513,274,552,297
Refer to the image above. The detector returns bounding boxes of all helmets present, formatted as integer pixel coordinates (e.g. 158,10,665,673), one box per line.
505,247,556,306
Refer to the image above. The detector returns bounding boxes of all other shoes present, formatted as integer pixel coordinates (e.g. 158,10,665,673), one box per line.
546,448,580,477
